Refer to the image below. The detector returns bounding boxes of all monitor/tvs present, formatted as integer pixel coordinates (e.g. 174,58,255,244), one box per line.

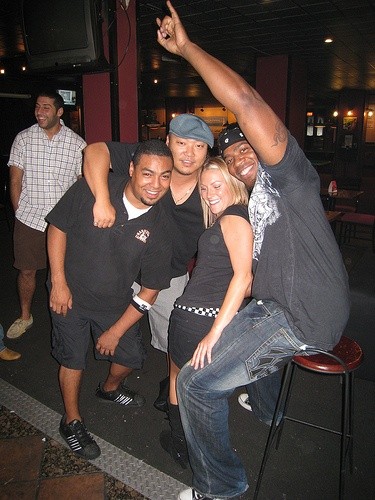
20,0,102,67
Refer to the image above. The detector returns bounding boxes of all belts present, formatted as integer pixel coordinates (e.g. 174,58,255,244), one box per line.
174,303,239,319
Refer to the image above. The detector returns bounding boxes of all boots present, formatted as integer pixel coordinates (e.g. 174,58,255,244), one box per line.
160,401,189,465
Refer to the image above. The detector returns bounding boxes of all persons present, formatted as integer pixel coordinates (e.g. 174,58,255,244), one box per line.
44,140,174,460
7,93,87,338
0,324,21,361
160,156,253,469
157,0,349,500
83,115,215,411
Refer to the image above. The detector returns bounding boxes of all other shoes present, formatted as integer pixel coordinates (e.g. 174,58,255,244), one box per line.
154,376,170,412
6,314,33,338
0,348,21,360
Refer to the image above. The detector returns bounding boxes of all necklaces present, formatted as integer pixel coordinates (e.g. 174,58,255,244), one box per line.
172,176,198,204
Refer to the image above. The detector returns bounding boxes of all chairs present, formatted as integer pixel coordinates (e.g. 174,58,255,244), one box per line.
338,213,375,253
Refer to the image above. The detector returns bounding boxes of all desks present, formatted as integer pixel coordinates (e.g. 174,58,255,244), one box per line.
320,187,366,249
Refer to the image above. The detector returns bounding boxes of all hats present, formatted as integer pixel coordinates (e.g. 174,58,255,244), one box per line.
216,123,246,160
168,113,214,149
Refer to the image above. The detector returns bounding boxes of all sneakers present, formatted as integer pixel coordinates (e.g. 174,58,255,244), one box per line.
238,393,252,412
58,413,101,459
95,382,145,407
178,487,224,500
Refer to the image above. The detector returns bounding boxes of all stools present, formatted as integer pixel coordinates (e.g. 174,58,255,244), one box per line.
255,335,365,500
